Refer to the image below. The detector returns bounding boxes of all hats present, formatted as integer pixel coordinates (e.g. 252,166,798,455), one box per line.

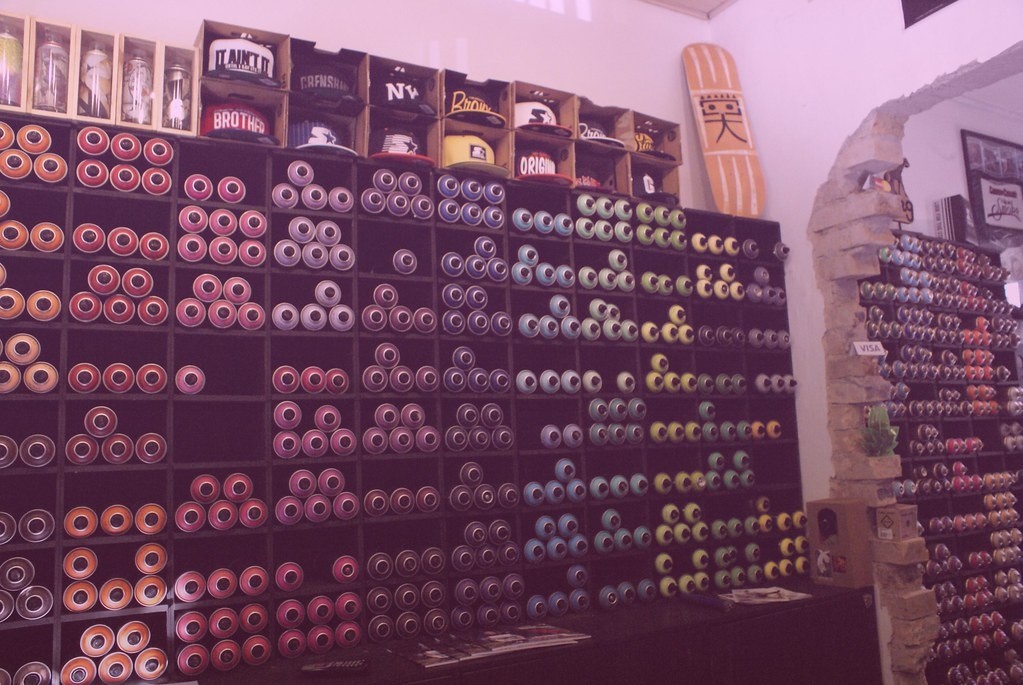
577,118,625,149
206,37,282,87
293,60,352,101
370,75,437,115
514,149,574,187
445,85,506,128
287,119,358,157
515,101,574,139
633,171,679,205
576,166,614,192
368,125,434,167
633,132,677,160
441,134,509,178
200,101,280,146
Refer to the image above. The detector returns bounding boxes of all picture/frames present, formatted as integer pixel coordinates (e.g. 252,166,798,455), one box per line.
960,128,1023,248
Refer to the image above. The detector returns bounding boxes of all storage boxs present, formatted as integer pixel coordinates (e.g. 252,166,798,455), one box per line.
194,19,683,206
0,10,198,137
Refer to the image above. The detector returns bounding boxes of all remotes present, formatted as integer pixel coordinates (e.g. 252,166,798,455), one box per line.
682,593,735,611
301,657,373,676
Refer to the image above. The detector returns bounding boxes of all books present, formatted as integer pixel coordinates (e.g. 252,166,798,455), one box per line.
719,583,813,604
384,622,590,669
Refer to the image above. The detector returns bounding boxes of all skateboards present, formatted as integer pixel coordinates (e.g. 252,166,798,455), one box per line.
680,42,769,218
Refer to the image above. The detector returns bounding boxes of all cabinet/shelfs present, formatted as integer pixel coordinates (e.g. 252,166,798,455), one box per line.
860,228,1023,685
1,108,884,685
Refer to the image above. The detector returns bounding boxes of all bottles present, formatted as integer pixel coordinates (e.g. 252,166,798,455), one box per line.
76,42,113,119
0,21,23,106
162,56,192,131
33,32,69,114
121,48,152,125
